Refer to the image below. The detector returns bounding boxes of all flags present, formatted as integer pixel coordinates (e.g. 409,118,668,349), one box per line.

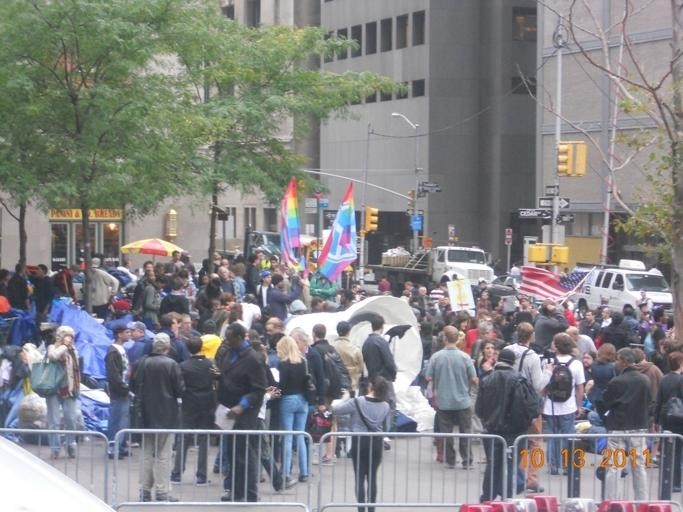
316,181,357,284
280,175,308,280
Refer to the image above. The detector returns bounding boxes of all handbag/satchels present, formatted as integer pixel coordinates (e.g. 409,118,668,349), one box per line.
368,426,383,452
303,373,318,404
133,395,145,429
306,410,332,442
659,396,683,423
30,361,66,398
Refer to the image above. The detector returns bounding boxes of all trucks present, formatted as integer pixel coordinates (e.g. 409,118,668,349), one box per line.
367,247,496,297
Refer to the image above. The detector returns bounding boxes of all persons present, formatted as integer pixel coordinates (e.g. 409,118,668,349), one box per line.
0,252,683,512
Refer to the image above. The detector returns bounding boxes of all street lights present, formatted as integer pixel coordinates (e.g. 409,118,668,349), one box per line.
391,111,422,250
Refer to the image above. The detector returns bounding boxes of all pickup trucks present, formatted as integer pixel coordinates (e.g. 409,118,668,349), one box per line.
220,231,278,264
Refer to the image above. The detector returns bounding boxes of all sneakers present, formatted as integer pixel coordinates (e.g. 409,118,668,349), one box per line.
51,442,129,460
140,452,353,502
435,457,557,492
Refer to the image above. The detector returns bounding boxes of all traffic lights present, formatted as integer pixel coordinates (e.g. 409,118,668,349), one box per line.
555,142,571,179
365,207,380,232
407,189,415,216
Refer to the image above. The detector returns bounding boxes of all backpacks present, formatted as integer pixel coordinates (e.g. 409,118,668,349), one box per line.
546,356,576,402
322,350,352,400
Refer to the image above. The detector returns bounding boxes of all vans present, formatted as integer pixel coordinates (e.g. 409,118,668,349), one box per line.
569,260,673,320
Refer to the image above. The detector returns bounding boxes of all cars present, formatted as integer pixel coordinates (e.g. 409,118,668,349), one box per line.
483,274,523,304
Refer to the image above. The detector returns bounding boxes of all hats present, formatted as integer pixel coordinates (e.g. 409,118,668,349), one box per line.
498,349,515,366
260,271,271,282
113,322,170,349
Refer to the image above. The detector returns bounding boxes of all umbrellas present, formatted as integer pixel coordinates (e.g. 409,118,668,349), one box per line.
120,239,185,266
